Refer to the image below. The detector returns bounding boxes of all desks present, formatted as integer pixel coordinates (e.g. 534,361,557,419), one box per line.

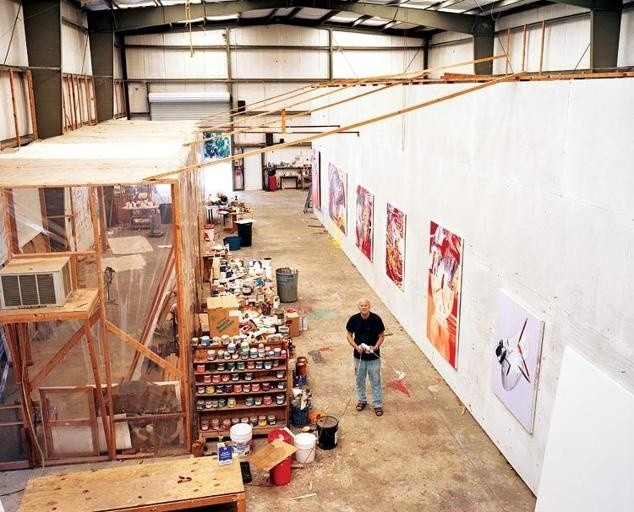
122,205,160,231
18,453,246,512
280,175,298,189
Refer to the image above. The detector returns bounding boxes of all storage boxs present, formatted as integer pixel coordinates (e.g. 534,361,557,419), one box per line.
207,294,240,338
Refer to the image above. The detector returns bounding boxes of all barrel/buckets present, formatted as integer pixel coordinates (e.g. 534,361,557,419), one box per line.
270,455,294,485
227,236,241,251
317,416,339,450
237,222,252,246
276,268,298,302
291,399,313,427
231,423,253,457
294,432,317,465
267,428,292,447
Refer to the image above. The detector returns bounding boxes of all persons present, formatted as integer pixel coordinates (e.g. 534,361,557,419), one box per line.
346,299,385,416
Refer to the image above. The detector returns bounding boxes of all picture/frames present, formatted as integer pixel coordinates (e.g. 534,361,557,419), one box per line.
328,160,348,236
426,219,464,367
355,184,375,263
311,148,321,209
385,201,407,292
489,287,546,436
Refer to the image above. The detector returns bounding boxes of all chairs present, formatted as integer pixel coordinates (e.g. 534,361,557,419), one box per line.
301,164,312,191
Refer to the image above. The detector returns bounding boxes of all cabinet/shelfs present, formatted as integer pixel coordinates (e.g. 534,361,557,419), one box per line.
0,254,74,309
191,340,290,442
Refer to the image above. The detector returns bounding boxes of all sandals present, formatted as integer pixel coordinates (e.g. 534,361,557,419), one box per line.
374,408,384,417
356,401,367,411
